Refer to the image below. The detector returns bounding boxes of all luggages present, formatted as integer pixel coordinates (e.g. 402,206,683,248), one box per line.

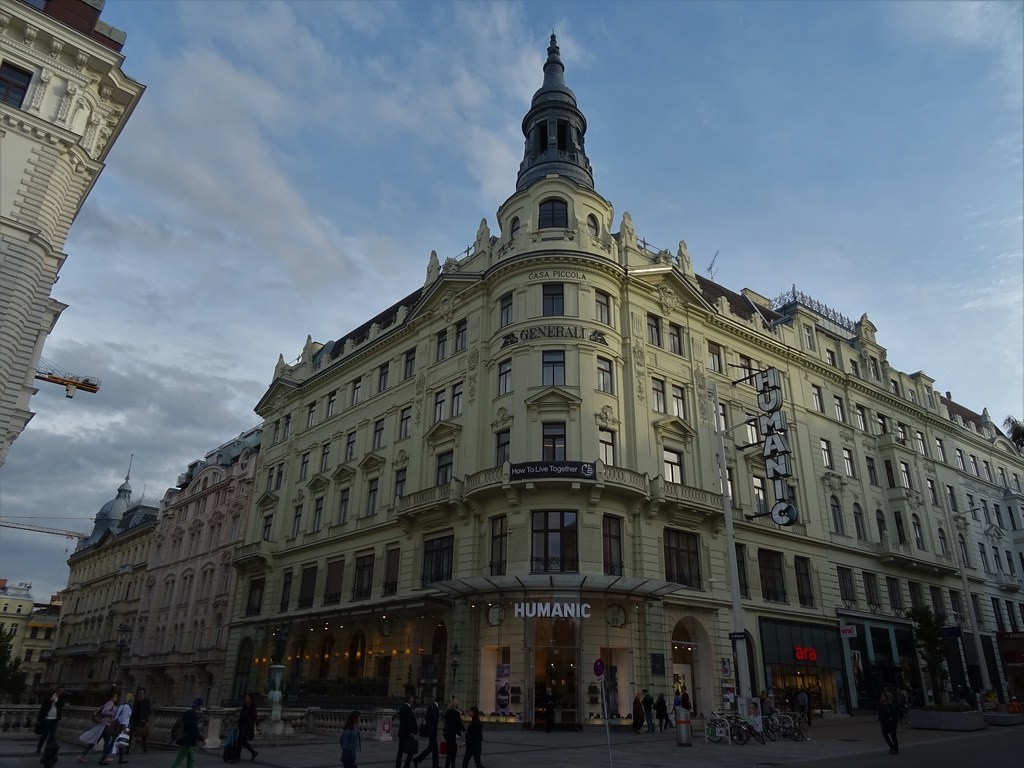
223,727,238,762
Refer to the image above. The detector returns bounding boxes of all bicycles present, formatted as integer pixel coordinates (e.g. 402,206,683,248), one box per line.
705,710,811,745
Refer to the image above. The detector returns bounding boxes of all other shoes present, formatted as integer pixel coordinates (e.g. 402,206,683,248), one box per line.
119,757,128,763
80,758,88,762
99,760,109,765
251,753,258,761
413,758,418,768
230,758,239,764
888,744,898,754
104,757,112,761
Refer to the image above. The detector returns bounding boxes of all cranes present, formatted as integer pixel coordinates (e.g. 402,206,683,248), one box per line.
0,515,96,554
35,357,102,400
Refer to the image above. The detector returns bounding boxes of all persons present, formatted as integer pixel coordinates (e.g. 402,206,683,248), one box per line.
797,683,912,724
396,695,419,768
441,700,466,768
878,694,899,755
655,692,669,733
412,695,445,768
462,706,486,768
632,695,645,733
497,683,509,708
125,688,151,753
748,702,761,718
35,685,67,754
642,689,656,734
99,692,133,764
77,691,118,762
338,710,361,768
231,693,261,762
169,698,206,767
680,686,690,712
541,688,557,731
671,690,681,714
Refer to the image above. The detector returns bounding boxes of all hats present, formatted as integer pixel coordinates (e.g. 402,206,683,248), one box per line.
191,698,203,708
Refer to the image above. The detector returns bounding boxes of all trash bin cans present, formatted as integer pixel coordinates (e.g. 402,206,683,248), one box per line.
675,706,692,747
971,691,981,711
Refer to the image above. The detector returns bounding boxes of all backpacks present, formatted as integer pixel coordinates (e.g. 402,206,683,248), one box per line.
93,705,113,723
172,714,194,740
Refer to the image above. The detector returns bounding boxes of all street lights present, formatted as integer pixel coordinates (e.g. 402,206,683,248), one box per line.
948,506,992,690
111,623,135,695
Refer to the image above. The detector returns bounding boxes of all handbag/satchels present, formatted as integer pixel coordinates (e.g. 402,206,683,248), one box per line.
115,727,130,747
403,738,418,754
653,703,657,709
419,723,429,737
111,720,120,733
135,722,149,736
79,722,105,746
35,719,43,734
440,738,449,754
